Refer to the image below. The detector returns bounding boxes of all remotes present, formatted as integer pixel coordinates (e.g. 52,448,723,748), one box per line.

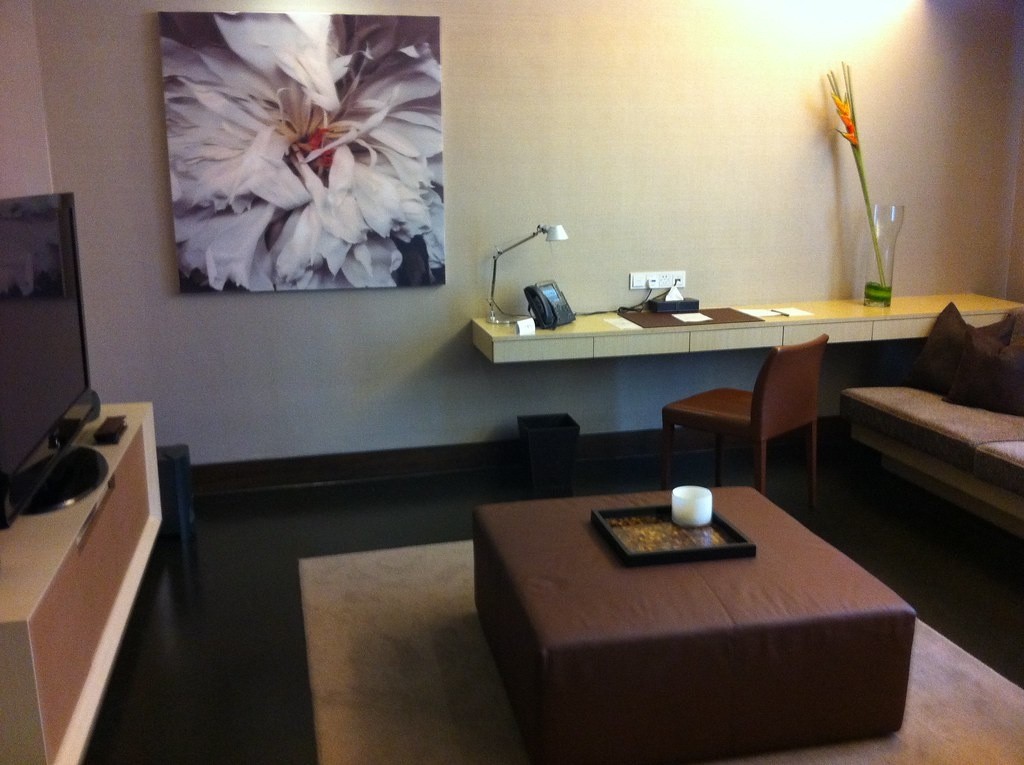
94,415,127,445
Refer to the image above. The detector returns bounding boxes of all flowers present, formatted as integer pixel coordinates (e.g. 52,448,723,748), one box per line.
826,61,888,289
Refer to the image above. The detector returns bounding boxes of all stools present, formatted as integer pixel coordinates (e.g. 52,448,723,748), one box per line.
517,414,581,498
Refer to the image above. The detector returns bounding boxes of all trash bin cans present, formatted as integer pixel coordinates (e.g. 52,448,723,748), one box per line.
518,413,580,500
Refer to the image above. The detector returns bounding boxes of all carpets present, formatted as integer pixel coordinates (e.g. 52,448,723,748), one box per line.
299,541,1024,765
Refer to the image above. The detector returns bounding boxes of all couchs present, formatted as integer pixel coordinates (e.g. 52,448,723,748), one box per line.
837,309,1024,536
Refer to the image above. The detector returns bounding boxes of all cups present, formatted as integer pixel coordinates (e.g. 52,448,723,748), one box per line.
671,485,712,527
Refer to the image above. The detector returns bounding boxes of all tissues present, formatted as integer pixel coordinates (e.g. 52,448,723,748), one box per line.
649,286,699,313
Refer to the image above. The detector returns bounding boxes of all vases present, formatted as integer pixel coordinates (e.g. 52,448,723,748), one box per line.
862,203,905,309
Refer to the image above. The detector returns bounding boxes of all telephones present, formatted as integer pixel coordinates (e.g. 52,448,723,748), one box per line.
524,280,576,329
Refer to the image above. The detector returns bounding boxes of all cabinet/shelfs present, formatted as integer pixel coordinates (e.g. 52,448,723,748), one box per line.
470,295,1024,363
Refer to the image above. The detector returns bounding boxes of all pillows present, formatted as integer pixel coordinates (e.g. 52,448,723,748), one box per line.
912,302,973,394
942,328,1024,416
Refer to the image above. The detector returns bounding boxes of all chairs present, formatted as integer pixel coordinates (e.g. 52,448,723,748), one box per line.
661,334,829,510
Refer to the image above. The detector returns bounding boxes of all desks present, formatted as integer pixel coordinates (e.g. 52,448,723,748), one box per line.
474,487,916,765
0,401,162,765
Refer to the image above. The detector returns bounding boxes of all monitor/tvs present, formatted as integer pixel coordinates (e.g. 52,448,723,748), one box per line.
0,191,109,530
